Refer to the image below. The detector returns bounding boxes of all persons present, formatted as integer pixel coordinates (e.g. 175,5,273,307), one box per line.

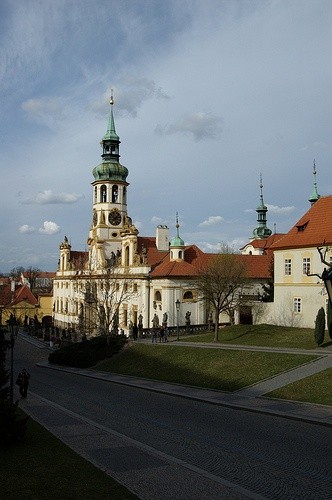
159,326,168,343
133,323,138,341
138,314,143,325
18,368,31,401
163,312,167,322
185,311,191,320
152,313,159,328
151,328,156,343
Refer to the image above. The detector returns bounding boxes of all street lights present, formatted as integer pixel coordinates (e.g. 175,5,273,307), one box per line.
175,298,181,341
5,313,18,407
